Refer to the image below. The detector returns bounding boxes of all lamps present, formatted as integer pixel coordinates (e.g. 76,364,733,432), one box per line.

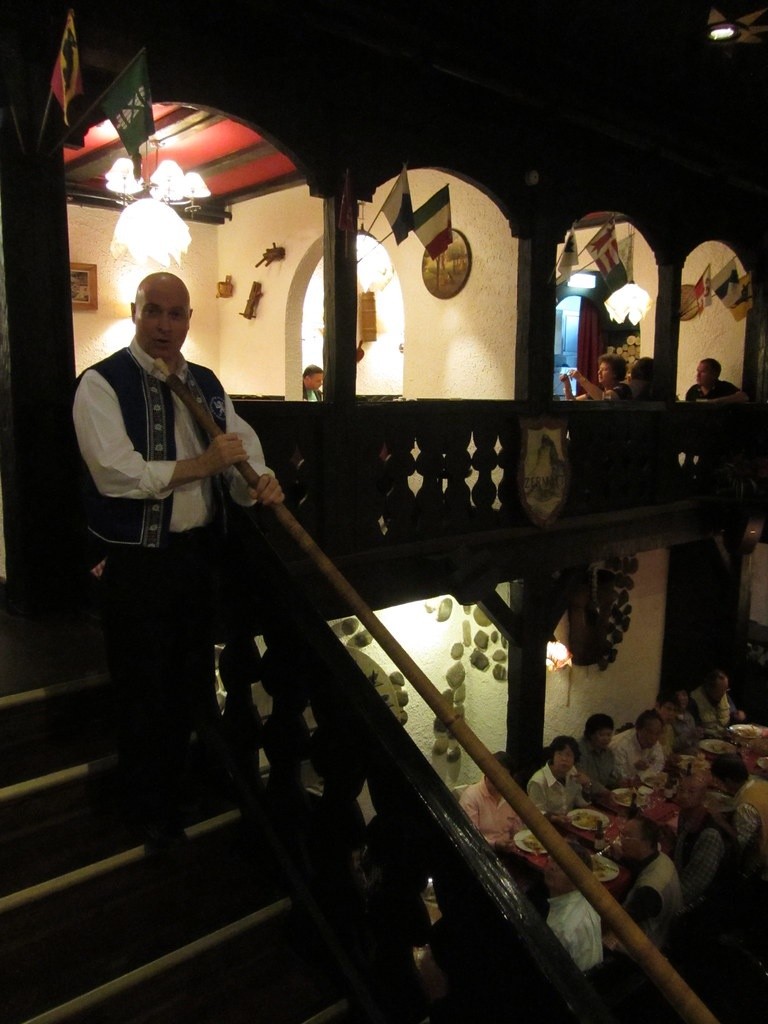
698,0,768,58
106,139,211,267
604,222,650,325
545,634,572,671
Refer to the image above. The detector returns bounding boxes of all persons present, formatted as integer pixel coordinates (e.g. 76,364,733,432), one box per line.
302,364,324,402
560,352,654,402
70,270,287,834
686,358,749,401
459,671,768,972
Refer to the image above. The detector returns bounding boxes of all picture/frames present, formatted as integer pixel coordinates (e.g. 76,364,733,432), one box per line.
69,262,98,309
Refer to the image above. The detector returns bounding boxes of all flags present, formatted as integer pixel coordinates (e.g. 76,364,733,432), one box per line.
380,165,415,242
726,272,753,322
338,174,358,234
49,14,81,122
98,50,154,156
413,185,452,259
558,227,579,283
695,264,712,320
711,259,740,307
618,236,635,283
586,217,620,279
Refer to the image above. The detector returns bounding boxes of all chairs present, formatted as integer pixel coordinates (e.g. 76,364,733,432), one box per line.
667,821,743,949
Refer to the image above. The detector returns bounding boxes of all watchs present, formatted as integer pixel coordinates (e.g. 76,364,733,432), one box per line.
585,380,589,382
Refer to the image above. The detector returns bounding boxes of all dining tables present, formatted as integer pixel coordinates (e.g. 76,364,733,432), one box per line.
495,724,768,932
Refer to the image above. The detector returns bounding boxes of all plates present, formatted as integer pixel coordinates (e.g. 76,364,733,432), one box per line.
676,755,711,773
699,739,737,754
730,724,764,738
567,808,610,830
590,854,620,882
703,791,735,813
514,829,548,853
639,770,668,788
757,757,768,770
612,786,655,807
